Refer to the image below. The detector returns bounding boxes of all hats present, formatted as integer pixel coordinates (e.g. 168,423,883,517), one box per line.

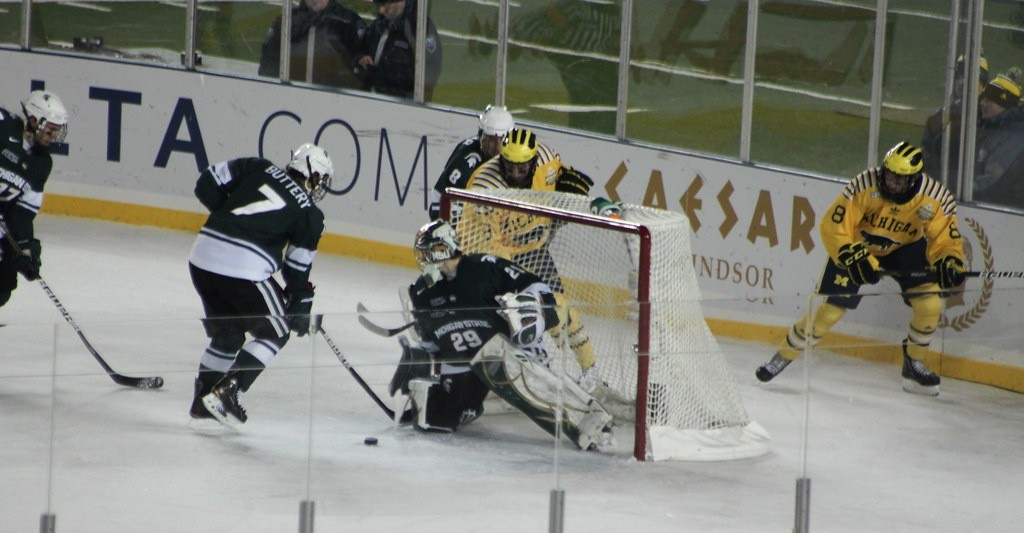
984,75,1021,112
955,50,988,89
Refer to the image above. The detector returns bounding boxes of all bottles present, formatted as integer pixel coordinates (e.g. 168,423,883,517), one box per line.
588,197,622,220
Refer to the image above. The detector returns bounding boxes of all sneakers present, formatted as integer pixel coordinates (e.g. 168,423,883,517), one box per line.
202,362,248,432
190,376,239,436
900,337,940,397
592,420,615,452
755,351,793,385
580,368,609,396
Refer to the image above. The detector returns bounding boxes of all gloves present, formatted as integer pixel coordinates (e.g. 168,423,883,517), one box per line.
929,254,966,290
284,281,315,336
837,242,882,286
554,167,595,198
11,237,41,281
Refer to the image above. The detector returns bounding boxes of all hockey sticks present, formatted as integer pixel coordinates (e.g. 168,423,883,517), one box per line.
355,314,416,337
879,267,1024,279
36,274,164,390
319,325,413,423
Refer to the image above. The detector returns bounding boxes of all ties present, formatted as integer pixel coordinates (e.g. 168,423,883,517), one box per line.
373,20,394,67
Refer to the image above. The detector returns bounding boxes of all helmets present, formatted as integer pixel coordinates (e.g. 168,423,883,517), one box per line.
414,218,462,276
879,140,922,201
478,104,514,159
287,143,334,204
20,90,67,153
500,128,538,187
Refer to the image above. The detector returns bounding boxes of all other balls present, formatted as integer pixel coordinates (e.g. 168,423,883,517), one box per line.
364,437,378,445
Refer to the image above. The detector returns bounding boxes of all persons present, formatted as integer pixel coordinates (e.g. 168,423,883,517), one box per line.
349,0,443,102
428,103,516,232
921,46,1024,210
389,217,618,455
475,0,644,135
452,128,607,395
754,142,969,397
189,141,334,432
0,90,69,327
258,0,371,93
